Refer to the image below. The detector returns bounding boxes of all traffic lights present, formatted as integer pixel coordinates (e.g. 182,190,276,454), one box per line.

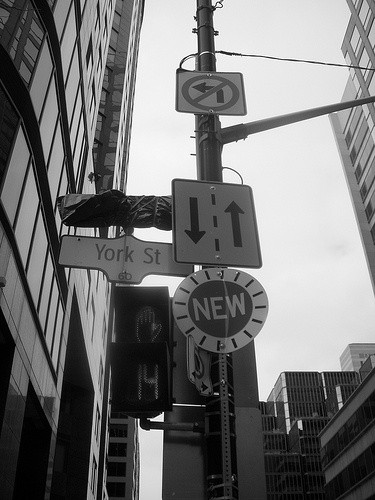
106,342,175,414
109,285,174,348
